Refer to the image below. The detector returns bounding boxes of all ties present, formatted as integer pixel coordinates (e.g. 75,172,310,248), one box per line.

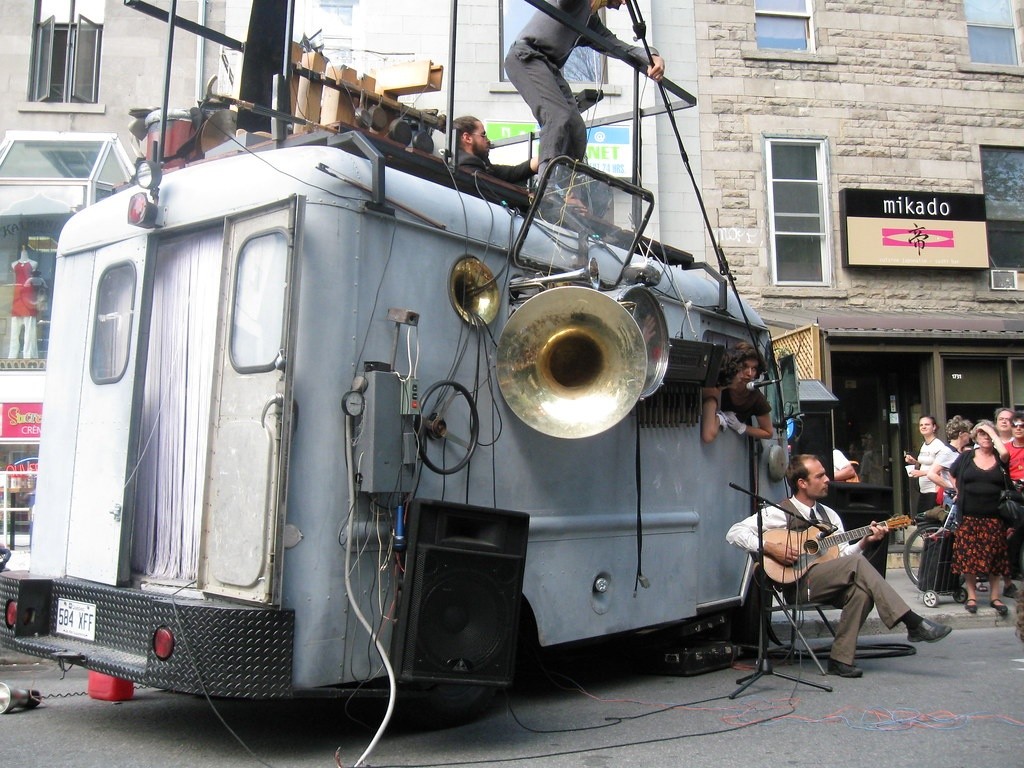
810,509,820,524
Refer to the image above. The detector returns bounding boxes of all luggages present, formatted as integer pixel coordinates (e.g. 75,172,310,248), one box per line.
917,500,967,607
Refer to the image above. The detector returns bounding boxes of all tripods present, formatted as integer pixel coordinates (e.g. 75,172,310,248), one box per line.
730,482,833,699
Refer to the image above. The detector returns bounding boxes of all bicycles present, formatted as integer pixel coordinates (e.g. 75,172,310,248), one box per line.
903,489,966,592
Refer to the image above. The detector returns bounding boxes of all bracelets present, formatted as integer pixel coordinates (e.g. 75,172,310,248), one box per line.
994,439,1002,449
992,434,998,443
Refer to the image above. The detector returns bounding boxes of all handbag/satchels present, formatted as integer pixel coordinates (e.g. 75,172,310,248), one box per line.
998,489,1024,528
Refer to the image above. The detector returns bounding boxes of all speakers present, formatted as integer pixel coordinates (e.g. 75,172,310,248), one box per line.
391,497,531,691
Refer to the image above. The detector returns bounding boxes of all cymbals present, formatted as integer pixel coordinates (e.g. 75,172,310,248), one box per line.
195,109,238,155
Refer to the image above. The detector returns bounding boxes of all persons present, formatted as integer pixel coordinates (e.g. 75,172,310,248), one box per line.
503,0,665,214
905,407,1024,614
832,447,856,482
8,250,38,359
726,454,952,678
701,340,774,444
455,117,538,185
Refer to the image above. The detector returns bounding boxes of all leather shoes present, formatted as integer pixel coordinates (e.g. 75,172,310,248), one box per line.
965,599,977,613
991,599,1007,613
828,657,863,677
906,618,952,643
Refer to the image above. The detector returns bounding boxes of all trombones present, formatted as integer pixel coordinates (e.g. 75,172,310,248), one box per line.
509,255,601,305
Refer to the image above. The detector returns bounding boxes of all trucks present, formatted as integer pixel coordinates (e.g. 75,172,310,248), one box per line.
0,127,804,727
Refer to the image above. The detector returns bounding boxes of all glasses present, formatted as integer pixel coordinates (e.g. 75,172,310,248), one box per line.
469,134,487,141
1011,422,1024,428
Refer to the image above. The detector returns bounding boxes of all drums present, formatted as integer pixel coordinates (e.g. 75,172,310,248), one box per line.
605,283,673,401
145,107,200,170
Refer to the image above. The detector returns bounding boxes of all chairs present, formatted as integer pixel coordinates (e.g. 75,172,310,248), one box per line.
749,551,842,676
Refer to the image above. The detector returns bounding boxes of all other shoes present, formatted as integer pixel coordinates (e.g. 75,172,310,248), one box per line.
1003,583,1018,598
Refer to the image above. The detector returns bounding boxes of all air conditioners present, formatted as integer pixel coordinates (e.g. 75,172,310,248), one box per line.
990,270,1018,291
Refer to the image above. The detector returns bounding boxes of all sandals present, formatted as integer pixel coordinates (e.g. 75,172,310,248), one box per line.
975,584,987,592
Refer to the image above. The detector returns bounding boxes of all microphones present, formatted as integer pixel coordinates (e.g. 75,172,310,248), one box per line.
815,527,838,541
393,493,406,552
745,380,781,391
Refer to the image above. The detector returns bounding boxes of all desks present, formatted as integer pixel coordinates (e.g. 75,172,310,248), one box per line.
0,488,34,550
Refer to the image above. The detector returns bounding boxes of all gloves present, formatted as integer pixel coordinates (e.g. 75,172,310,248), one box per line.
724,412,746,435
716,414,728,433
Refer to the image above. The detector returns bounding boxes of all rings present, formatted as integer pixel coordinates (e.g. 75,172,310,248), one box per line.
662,72,664,75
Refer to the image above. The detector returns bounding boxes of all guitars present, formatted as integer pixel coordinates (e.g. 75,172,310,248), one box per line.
763,513,913,587
